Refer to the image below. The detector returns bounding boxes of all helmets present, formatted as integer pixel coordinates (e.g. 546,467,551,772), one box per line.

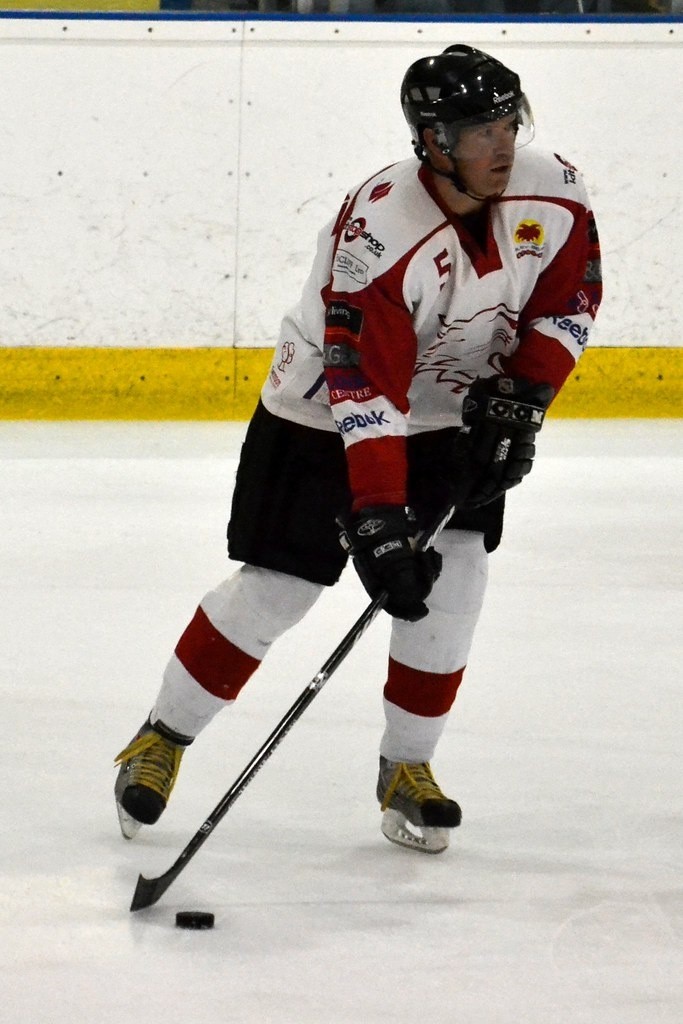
400,44,534,157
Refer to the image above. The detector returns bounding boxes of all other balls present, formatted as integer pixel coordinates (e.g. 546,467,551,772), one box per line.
175,911,215,931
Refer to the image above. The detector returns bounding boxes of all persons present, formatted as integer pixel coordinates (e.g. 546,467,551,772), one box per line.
113,43,604,855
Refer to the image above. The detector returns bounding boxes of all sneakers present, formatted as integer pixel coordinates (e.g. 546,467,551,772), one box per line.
376,754,462,855
113,708,195,839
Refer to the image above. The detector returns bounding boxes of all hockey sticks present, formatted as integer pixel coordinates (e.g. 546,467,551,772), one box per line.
127,503,457,914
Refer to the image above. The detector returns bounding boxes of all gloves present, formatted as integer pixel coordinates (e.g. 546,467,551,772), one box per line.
451,374,553,511
335,504,442,622
